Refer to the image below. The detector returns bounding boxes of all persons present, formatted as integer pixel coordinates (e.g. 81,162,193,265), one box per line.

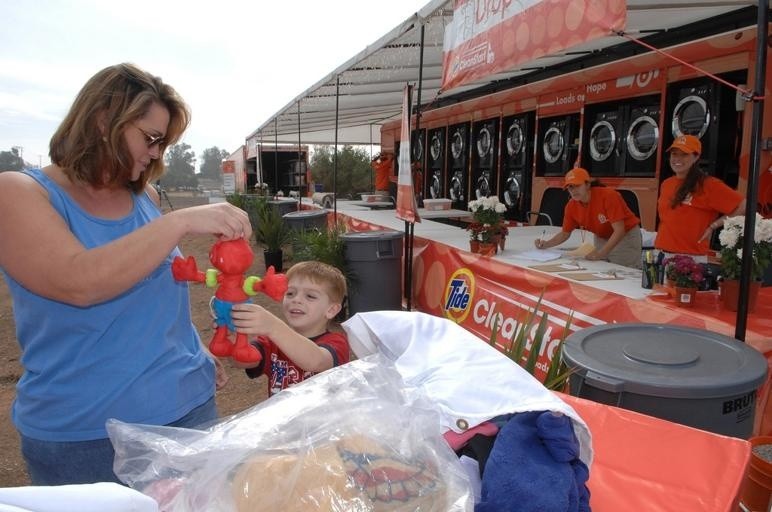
534,167,642,268
371,151,395,202
654,136,748,290
0,64,252,483
209,261,351,395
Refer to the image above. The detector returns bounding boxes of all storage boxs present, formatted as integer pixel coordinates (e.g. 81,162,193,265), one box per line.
423,198,451,210
360,194,382,202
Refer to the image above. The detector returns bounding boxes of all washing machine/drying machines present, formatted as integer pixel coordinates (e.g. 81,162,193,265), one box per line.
618,102,660,177
501,113,536,169
411,127,427,167
536,114,580,175
394,140,401,176
446,171,468,211
426,125,446,168
445,120,470,170
427,168,445,199
660,82,735,177
498,169,532,223
468,170,497,204
470,113,500,169
581,109,622,176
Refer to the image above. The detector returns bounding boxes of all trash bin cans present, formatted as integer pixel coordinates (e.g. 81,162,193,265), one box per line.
266,201,297,221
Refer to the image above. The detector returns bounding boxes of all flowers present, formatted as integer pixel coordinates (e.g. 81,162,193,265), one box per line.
480,196,509,241
675,256,703,287
719,212,772,280
467,196,487,239
663,255,677,280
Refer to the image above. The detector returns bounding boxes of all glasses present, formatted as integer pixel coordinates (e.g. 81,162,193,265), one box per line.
131,124,168,150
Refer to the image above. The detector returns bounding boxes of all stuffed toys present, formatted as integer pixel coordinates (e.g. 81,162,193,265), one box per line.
173,238,287,369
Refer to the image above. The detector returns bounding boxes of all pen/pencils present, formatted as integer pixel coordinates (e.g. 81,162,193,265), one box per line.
538,229,546,248
645,250,664,289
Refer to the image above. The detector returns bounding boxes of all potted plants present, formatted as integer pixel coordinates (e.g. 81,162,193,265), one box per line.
251,200,292,273
479,242,495,256
294,222,347,320
470,239,480,252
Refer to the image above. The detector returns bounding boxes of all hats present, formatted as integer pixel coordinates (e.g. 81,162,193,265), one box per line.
380,152,387,158
665,135,701,154
563,170,591,190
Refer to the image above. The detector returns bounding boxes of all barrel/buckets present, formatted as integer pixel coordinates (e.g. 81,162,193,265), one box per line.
739,435,772,512
267,200,298,235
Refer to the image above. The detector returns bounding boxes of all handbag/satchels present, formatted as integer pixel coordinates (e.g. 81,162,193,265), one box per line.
339,311,594,468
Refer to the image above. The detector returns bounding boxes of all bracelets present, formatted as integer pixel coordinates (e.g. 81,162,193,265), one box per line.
710,221,718,231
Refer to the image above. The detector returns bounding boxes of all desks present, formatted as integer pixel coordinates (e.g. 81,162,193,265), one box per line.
342,208,471,310
414,225,772,439
302,198,393,233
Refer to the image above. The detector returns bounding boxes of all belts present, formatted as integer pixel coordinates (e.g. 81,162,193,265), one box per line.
377,188,390,191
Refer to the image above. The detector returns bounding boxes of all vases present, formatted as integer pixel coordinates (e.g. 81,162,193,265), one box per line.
723,279,762,313
667,279,674,295
673,286,695,307
717,280,723,300
